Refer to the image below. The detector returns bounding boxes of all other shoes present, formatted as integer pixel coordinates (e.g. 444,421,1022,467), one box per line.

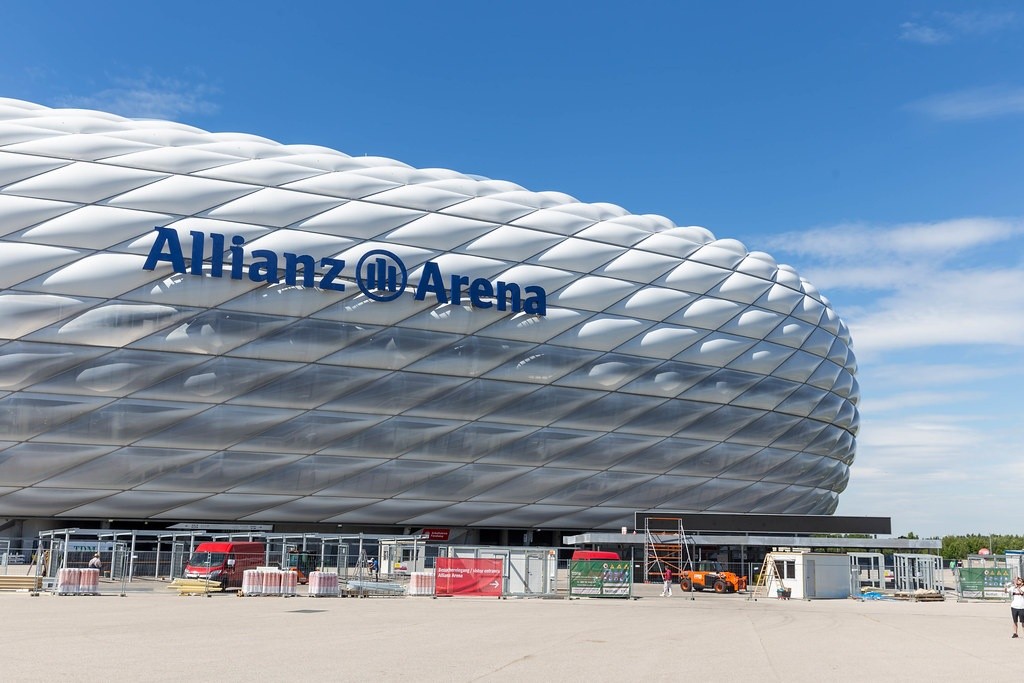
668,594,671,597
1012,634,1018,638
660,593,665,597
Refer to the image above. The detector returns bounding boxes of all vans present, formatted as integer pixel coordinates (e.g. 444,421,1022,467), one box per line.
182,541,264,593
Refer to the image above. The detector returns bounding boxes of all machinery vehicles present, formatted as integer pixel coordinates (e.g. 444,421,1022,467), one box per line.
287,549,324,584
679,561,749,594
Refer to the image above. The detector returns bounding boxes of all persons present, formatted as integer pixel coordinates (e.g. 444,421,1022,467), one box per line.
950,560,956,576
371,558,378,579
40,551,49,576
660,565,673,597
1005,578,1024,638
88,554,102,569
